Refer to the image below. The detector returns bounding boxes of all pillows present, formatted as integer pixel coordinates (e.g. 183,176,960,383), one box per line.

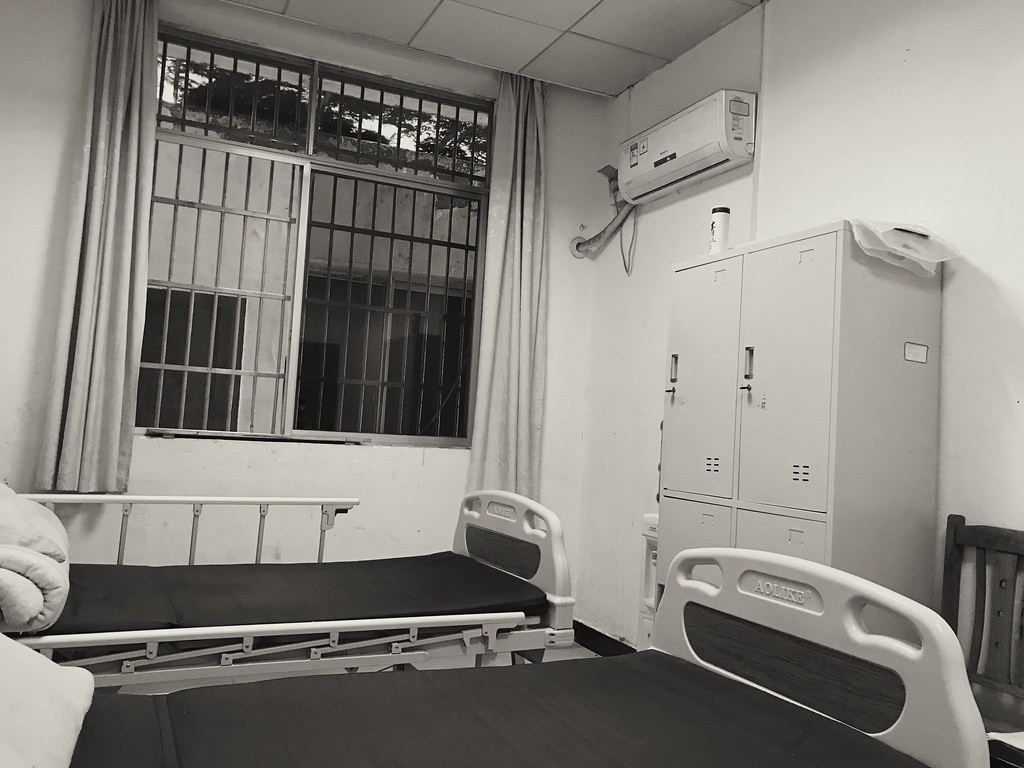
0,630,96,768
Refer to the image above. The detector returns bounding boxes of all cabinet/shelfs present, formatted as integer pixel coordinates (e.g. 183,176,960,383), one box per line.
653,218,945,628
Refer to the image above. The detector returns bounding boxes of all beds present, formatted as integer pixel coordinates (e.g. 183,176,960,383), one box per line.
1,546,990,768
0,481,578,690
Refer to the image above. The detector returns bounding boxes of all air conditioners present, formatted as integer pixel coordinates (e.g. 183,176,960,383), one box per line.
616,88,757,207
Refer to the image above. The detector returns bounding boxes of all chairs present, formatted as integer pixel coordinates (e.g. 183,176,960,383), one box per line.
933,514,1023,768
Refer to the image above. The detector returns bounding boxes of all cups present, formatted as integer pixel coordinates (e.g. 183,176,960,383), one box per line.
709,205,730,253
